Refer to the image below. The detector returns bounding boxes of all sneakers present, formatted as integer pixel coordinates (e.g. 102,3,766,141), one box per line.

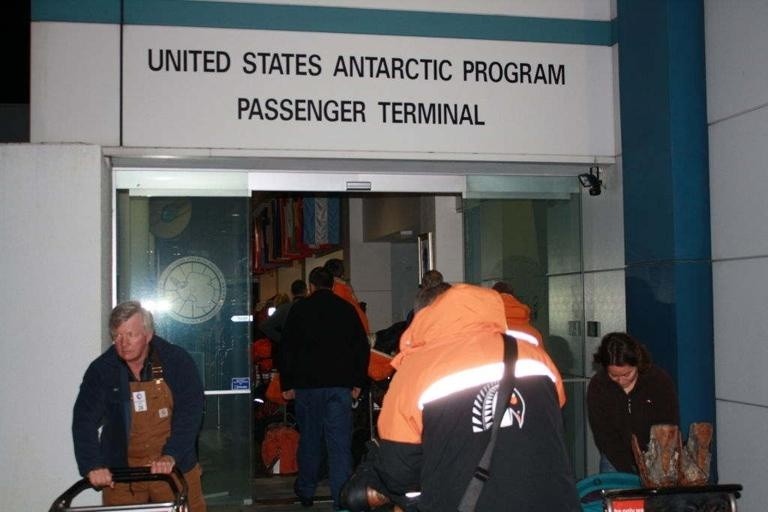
292,479,314,508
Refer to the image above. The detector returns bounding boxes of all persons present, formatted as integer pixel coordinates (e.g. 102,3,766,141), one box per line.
71,299,207,512
586,331,681,474
253,277,414,456
540,333,577,378
492,282,513,296
337,269,584,511
276,266,371,508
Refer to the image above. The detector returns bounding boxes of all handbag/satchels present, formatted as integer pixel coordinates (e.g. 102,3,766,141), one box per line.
260,424,301,477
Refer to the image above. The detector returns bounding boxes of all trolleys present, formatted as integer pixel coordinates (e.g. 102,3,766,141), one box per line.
593,482,749,512
49,464,190,512
